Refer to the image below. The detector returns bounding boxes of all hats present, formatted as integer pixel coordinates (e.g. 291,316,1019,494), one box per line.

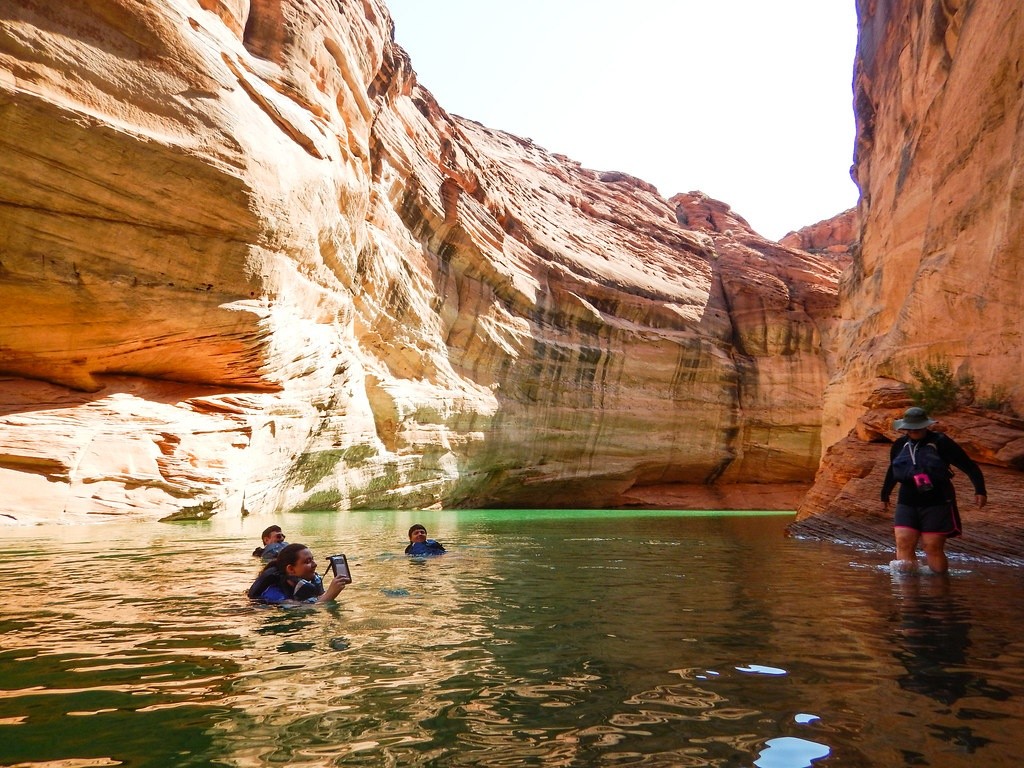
893,407,939,431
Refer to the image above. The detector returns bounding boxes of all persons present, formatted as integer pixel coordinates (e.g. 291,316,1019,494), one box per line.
261,525,285,545
404,524,445,554
262,544,345,603
881,407,986,571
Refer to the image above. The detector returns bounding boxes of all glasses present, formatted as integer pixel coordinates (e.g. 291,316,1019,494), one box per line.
276,534,285,538
